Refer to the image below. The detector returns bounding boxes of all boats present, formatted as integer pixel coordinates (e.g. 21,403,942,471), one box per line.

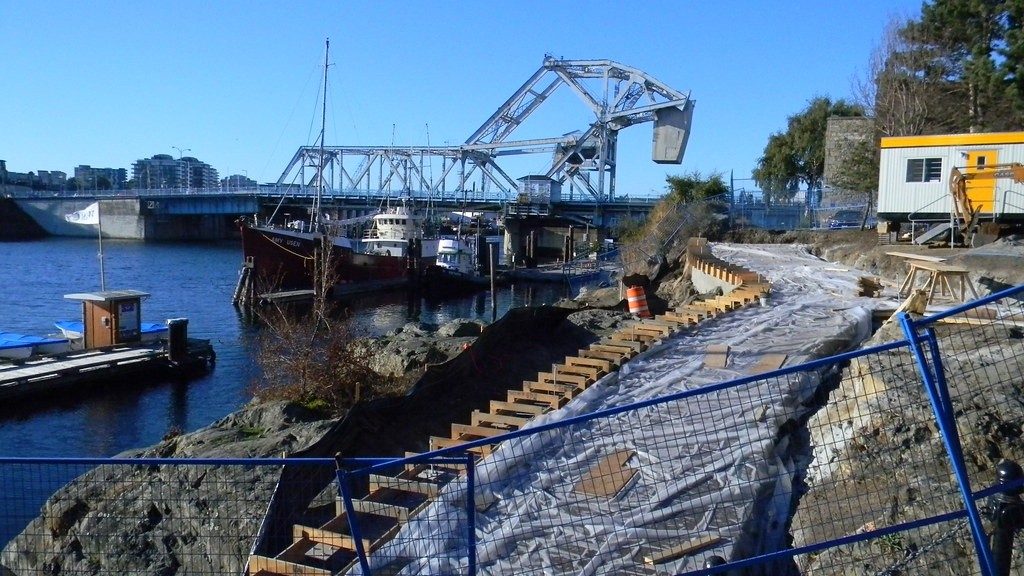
234,204,479,307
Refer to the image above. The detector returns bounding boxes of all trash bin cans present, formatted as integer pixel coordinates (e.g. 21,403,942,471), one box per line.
165,317,189,361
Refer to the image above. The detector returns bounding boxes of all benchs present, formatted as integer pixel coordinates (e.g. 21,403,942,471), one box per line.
886,250,947,297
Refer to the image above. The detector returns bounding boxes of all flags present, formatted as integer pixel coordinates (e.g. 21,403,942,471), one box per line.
65,201,100,225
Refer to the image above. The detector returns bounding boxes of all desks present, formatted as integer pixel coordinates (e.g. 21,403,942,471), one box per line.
896,259,969,306
